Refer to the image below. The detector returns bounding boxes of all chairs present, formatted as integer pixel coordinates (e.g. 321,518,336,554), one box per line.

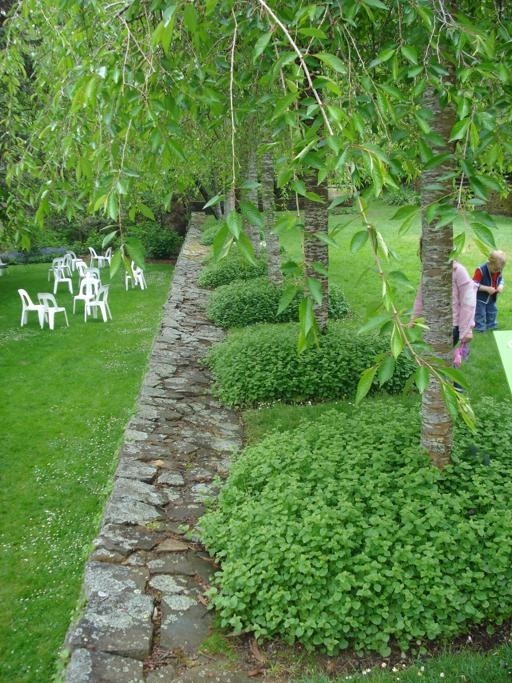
18,247,148,330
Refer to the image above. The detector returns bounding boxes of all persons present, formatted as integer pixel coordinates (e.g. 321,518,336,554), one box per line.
472,250,507,332
408,237,477,392
